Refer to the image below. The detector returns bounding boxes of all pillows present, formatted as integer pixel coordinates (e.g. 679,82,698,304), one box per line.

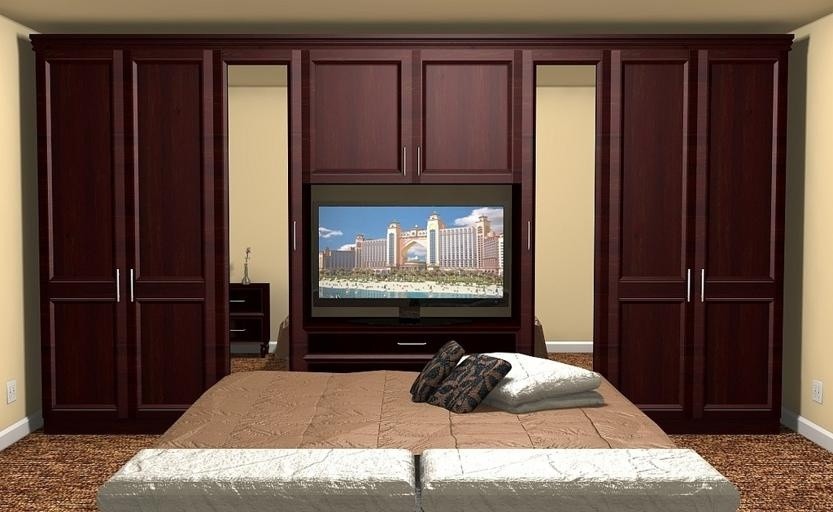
408,339,511,414
97,448,740,508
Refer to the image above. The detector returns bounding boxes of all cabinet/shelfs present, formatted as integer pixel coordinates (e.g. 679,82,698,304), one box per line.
27,32,231,436
230,282,271,359
594,32,795,436
285,32,536,370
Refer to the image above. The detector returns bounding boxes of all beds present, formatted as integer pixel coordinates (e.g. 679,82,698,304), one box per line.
95,350,742,510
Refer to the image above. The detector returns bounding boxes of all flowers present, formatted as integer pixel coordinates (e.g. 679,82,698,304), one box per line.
244,247,251,283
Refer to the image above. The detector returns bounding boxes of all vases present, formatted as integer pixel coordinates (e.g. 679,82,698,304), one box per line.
242,263,251,285
